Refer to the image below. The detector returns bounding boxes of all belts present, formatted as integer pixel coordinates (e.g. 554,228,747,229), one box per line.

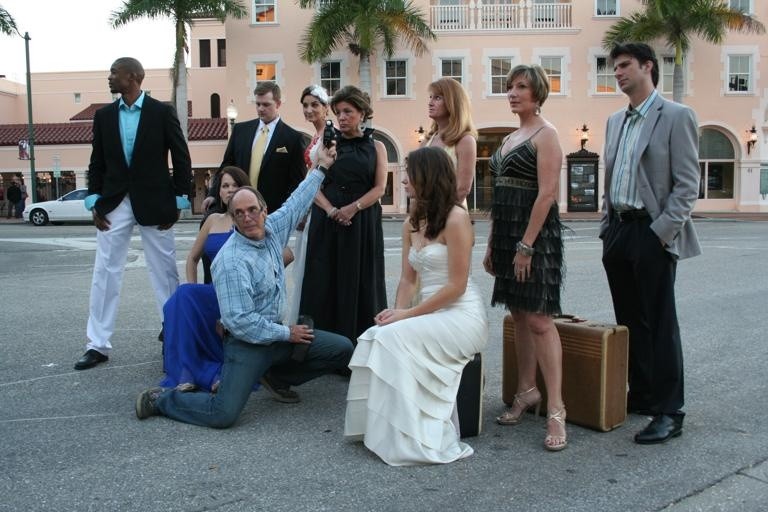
612,209,647,221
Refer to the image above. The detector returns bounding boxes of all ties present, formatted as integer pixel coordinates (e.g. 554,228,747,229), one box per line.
248,126,268,190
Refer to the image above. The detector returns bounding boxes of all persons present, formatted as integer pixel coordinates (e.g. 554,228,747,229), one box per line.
72,56,194,371
413,78,481,219
0,178,28,219
297,86,394,377
288,83,332,325
596,43,710,444
341,145,490,468
202,81,306,233
129,125,361,431
481,62,575,455
156,163,297,395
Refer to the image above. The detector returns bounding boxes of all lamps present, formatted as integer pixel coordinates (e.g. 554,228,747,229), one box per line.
746,125,758,155
579,125,589,150
414,127,424,143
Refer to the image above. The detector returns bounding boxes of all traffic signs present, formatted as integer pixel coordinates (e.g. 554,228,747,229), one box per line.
226,95,237,133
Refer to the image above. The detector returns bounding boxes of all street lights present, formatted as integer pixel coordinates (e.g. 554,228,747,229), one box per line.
1,8,38,202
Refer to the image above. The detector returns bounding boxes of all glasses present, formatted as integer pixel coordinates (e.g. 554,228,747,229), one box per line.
231,206,263,222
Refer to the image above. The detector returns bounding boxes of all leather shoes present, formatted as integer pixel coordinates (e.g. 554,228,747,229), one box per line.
636,409,685,442
74,350,108,370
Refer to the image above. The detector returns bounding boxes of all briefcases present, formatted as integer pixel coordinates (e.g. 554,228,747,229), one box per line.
503,313,630,432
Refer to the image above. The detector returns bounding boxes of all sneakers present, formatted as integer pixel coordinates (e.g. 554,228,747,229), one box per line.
136,386,168,420
260,371,301,404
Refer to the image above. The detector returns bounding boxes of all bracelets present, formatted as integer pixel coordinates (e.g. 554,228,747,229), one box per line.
513,240,536,258
315,165,329,175
355,199,364,212
326,206,338,219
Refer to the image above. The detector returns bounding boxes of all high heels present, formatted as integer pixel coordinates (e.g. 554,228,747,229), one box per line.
497,387,542,426
545,404,567,451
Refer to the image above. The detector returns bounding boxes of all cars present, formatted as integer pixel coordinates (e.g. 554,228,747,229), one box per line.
20,187,93,225
